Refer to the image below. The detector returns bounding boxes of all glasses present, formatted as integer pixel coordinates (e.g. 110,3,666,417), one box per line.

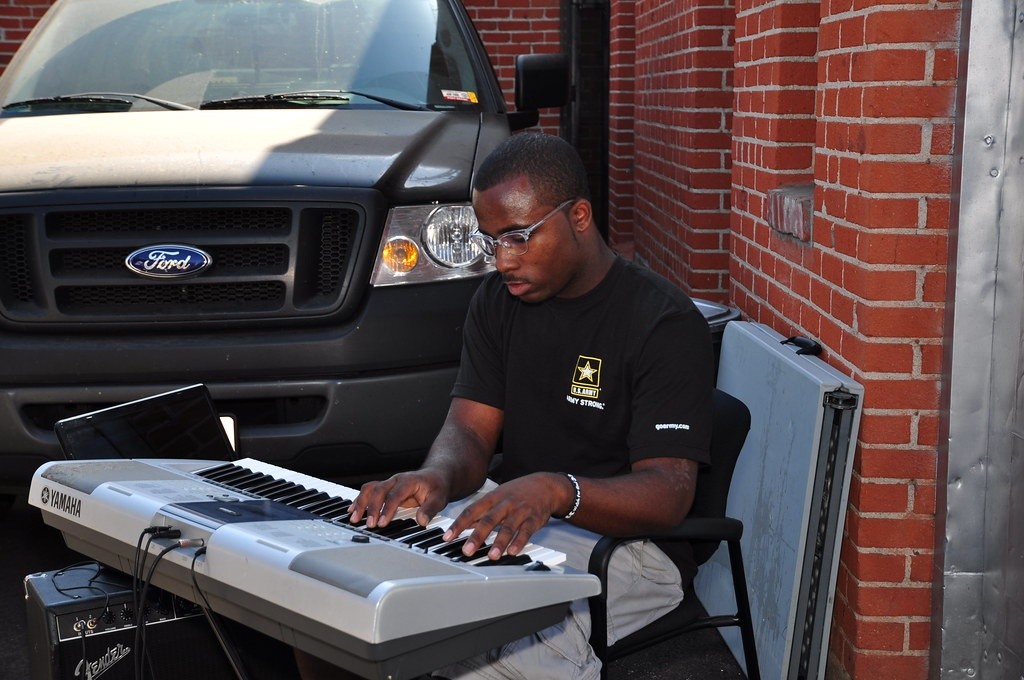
468,200,575,258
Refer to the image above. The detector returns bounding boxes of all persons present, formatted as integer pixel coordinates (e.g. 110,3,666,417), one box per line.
293,130,715,680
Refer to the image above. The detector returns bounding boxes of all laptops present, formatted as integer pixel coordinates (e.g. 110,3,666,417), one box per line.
53,383,238,463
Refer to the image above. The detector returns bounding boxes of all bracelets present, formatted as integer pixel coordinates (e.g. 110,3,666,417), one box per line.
551,472,581,520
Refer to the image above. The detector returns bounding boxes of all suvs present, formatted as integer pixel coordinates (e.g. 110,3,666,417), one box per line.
0,0,572,474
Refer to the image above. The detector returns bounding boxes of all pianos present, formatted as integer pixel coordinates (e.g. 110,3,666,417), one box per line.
28,454,608,678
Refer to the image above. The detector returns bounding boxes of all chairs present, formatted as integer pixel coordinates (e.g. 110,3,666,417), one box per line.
589,388,761,680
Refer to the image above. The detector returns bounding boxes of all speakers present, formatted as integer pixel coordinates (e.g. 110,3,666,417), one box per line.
23,563,304,680
